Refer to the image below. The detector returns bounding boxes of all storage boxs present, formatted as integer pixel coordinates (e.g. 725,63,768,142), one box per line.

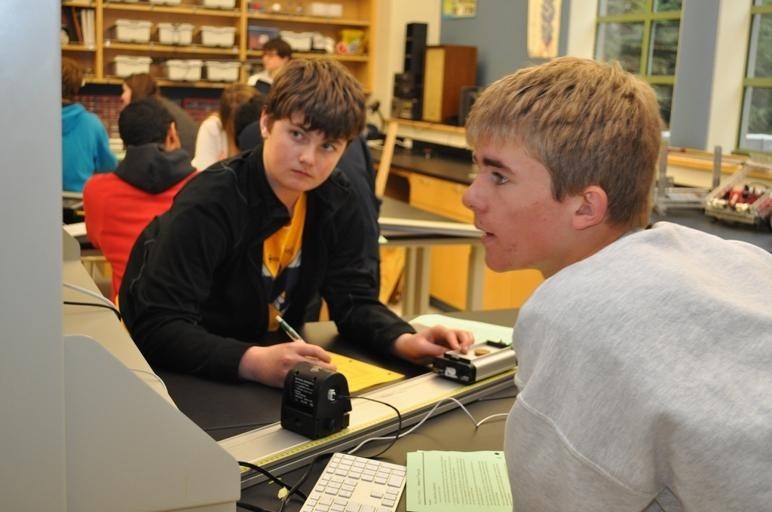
111,0,343,83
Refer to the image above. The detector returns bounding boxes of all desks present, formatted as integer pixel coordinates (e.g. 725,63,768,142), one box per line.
364,189,492,320
364,148,547,313
646,182,772,256
144,305,519,509
62,213,113,285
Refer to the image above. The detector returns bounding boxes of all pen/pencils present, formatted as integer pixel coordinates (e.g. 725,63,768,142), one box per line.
275,315,306,343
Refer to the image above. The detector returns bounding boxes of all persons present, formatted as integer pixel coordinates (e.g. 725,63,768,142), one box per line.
461,57,772,512
120,54,476,389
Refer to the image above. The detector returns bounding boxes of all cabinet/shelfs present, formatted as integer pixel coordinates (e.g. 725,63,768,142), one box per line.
59,0,378,95
420,43,478,126
404,21,427,120
